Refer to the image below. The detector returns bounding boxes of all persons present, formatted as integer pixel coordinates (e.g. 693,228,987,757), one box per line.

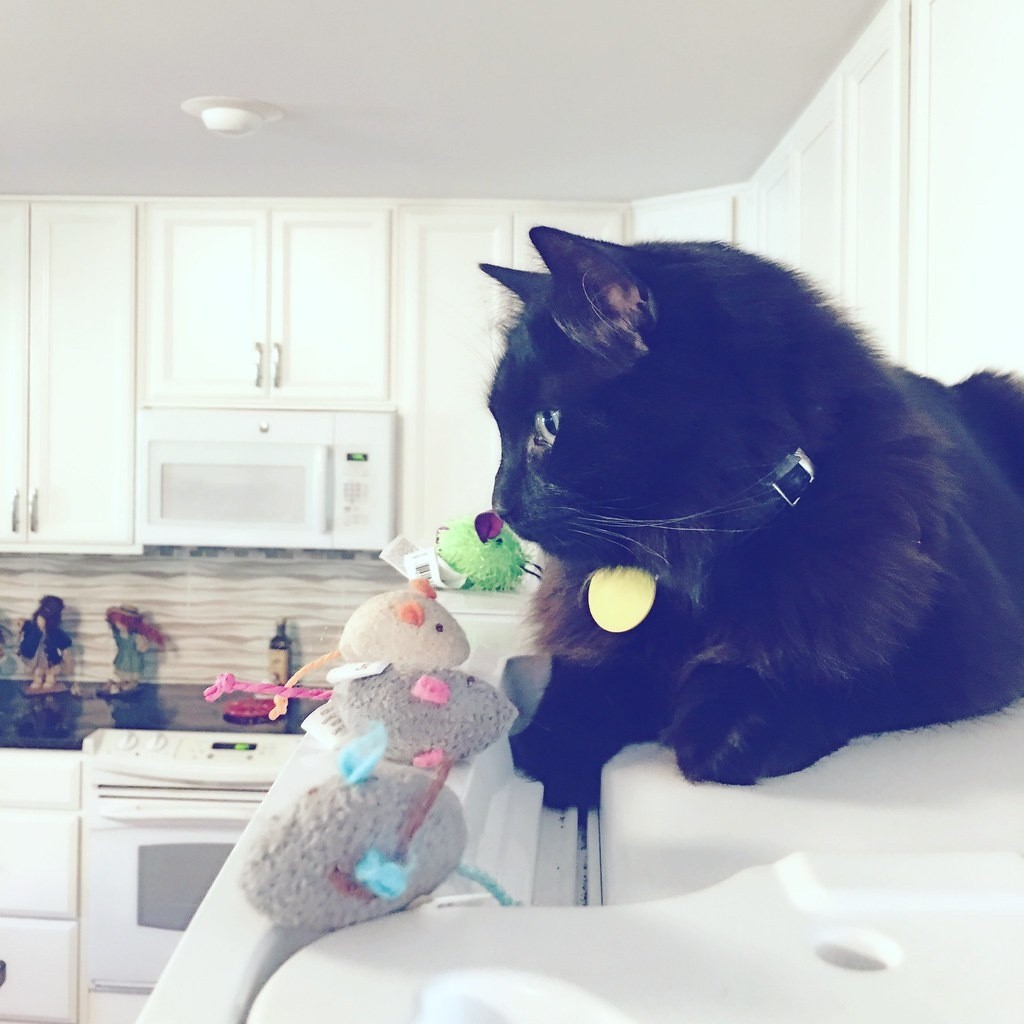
104,605,150,696
15,594,76,692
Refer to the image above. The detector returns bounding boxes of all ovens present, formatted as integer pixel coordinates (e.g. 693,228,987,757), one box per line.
82,727,306,1024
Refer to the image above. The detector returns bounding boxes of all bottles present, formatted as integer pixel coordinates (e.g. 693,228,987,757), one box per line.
269,623,293,685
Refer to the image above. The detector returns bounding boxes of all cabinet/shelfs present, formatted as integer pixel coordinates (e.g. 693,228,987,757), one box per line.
0,0,1024,1024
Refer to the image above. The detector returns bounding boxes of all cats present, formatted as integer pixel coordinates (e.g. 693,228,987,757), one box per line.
475,224,1024,814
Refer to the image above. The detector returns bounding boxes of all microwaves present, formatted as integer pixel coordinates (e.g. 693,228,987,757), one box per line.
139,407,395,551
